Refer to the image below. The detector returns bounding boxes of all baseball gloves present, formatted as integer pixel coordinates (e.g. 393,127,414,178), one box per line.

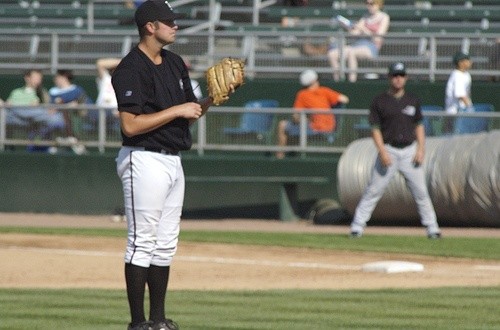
206,56,245,106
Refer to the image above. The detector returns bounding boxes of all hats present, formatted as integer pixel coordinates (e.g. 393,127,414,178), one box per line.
389,62,406,76
454,50,471,63
135,0,185,22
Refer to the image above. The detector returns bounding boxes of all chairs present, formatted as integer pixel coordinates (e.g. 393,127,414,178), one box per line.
420,105,445,135
454,104,495,134
353,118,369,138
304,101,344,145
218,99,279,157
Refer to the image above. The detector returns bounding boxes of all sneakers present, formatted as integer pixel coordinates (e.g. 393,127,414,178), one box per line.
127,318,179,330
349,232,363,239
428,233,440,240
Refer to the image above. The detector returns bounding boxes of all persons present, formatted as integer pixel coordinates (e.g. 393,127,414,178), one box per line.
42,67,95,154
109,0,243,330
1,69,67,152
444,52,475,115
93,57,125,130
347,61,443,241
274,69,349,159
328,0,390,83
